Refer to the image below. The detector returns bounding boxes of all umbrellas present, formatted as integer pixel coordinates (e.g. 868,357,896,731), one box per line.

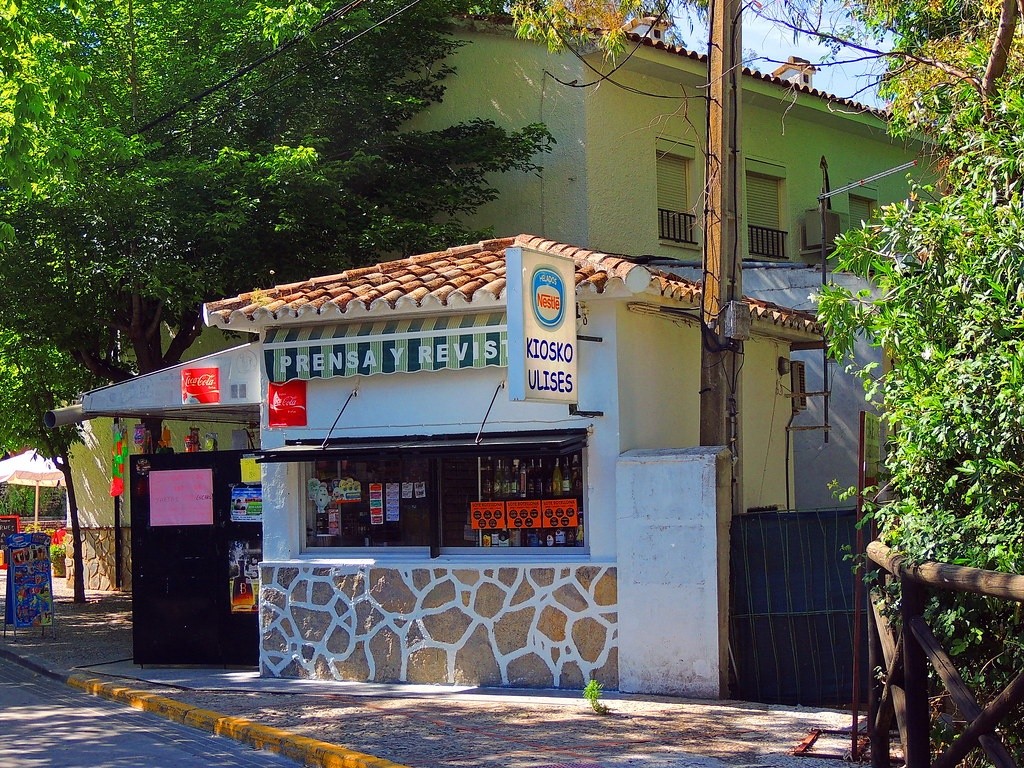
0,450,69,533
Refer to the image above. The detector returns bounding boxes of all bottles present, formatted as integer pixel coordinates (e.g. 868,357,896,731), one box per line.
482,509,584,546
482,455,581,498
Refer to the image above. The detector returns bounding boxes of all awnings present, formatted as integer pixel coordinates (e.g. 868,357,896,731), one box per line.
253,431,587,464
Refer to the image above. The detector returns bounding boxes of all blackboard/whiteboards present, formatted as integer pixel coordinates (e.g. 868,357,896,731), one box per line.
0,515,21,570
